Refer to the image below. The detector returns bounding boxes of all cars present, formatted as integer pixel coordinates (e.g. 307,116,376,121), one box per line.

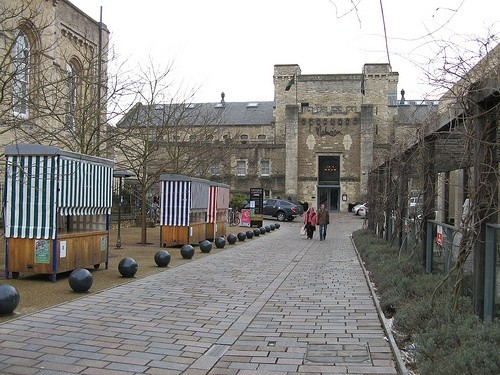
352,201,370,220
244,198,304,222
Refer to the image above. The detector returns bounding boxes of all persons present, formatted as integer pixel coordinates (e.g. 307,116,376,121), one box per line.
316,204,329,241
303,206,318,239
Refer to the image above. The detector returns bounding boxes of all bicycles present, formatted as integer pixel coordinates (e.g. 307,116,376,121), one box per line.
229,208,240,226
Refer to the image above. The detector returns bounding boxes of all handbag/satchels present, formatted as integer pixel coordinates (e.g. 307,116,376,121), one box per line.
300,224,306,235
